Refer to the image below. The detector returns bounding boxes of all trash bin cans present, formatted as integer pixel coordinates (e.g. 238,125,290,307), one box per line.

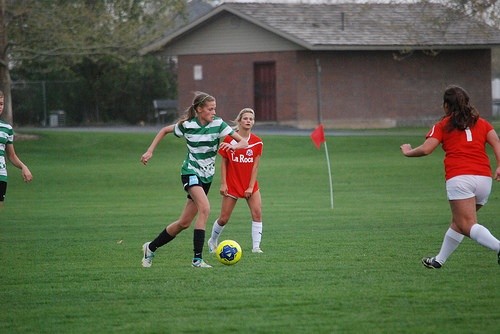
49,111,66,128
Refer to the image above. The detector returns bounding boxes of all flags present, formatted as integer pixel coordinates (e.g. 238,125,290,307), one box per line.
310,124,326,150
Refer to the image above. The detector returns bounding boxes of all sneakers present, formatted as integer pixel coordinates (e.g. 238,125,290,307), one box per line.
191,258,213,268
141,241,156,267
422,257,443,269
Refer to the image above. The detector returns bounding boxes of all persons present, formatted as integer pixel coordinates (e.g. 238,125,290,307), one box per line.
0,89,33,211
140,93,248,268
401,87,500,270
208,107,264,254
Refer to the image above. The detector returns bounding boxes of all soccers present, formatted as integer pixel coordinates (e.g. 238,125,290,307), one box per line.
215,239,242,265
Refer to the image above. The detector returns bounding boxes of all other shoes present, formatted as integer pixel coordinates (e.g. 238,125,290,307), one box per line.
252,248,264,254
207,238,217,254
